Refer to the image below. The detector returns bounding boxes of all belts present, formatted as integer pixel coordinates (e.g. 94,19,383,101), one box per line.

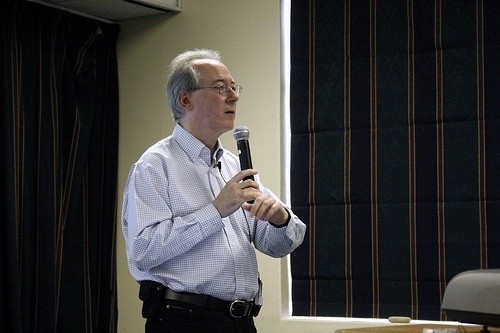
163,288,261,319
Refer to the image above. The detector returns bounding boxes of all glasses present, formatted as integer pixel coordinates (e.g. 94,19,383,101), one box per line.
187,85,243,96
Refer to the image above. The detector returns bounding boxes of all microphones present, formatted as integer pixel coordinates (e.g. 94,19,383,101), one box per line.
233,126,256,204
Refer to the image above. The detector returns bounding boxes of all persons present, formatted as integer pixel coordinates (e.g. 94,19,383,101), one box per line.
121,49,306,333
